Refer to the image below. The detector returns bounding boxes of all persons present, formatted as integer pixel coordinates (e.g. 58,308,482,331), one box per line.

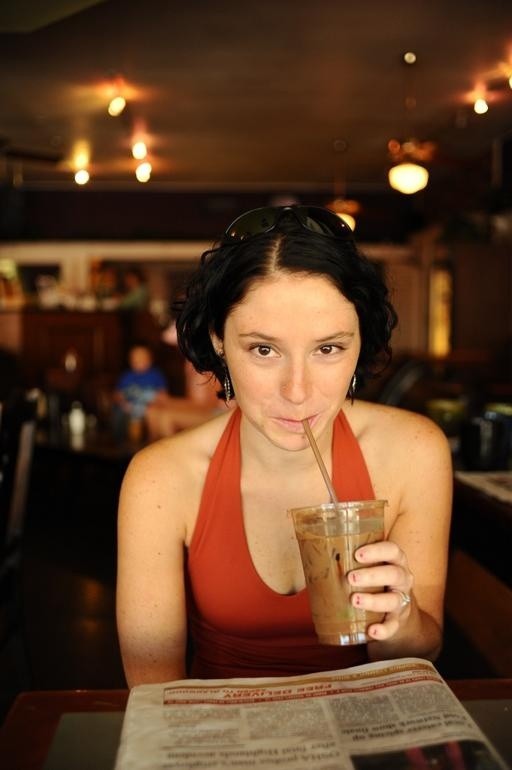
118,335,167,417
114,205,454,688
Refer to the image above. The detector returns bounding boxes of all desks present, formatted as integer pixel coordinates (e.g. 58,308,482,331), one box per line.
0,674,512,770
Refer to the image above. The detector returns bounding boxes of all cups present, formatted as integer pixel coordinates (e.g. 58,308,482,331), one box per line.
285,499,386,648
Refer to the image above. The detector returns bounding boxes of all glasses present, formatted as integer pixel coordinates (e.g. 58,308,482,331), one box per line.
225,205,352,243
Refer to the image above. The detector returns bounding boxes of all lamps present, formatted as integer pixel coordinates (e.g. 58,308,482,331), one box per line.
389,145,429,195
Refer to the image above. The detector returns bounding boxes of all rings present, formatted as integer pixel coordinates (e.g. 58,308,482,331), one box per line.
399,590,410,609
